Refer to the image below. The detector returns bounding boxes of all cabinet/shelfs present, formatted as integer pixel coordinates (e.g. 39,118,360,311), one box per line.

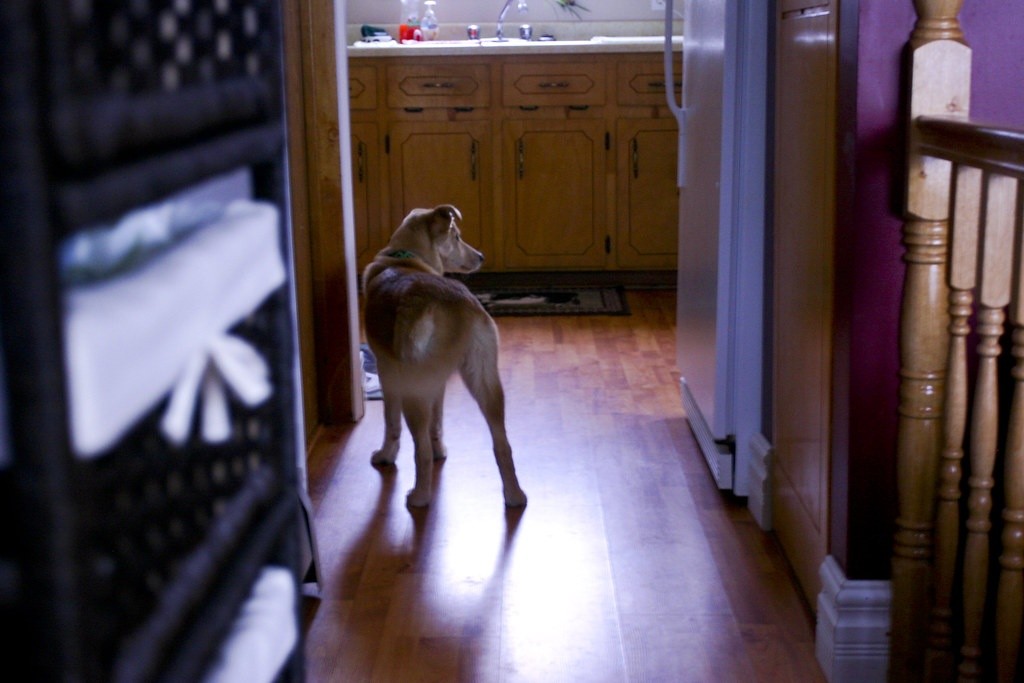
346,51,683,290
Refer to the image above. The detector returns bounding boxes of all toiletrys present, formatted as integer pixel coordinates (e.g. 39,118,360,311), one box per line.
420,1,439,41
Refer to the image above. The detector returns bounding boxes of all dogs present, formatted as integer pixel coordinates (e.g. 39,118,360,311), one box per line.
361,203,526,507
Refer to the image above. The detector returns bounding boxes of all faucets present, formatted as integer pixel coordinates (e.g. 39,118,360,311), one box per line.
496,0,528,38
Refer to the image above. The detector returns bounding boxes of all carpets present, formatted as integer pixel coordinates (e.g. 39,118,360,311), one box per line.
466,286,632,317
